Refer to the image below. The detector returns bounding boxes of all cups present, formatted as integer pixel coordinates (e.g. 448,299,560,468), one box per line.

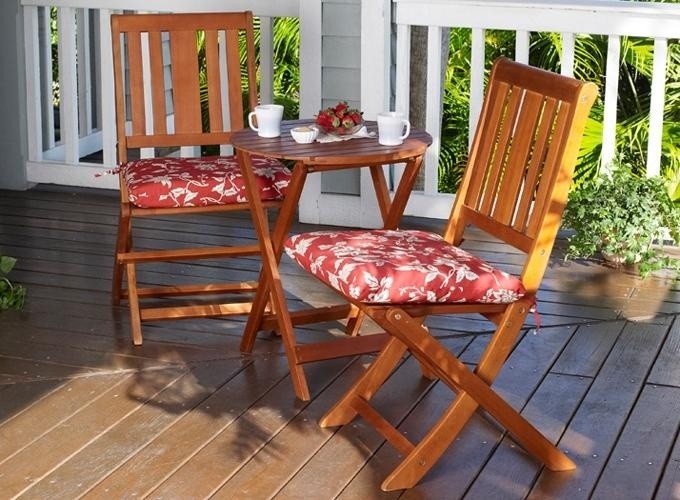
376,112,410,146
248,104,285,138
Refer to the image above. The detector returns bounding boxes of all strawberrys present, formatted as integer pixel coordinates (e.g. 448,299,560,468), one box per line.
315,103,363,135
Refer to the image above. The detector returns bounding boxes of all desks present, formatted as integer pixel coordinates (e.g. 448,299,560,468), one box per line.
226,120,447,403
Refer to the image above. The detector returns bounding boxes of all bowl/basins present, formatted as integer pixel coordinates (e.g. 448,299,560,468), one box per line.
316,120,365,137
290,127,319,143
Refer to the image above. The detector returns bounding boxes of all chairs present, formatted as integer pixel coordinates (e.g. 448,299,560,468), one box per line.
100,8,304,350
278,49,608,494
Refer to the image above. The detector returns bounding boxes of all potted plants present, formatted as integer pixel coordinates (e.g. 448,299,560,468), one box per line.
554,151,678,285
